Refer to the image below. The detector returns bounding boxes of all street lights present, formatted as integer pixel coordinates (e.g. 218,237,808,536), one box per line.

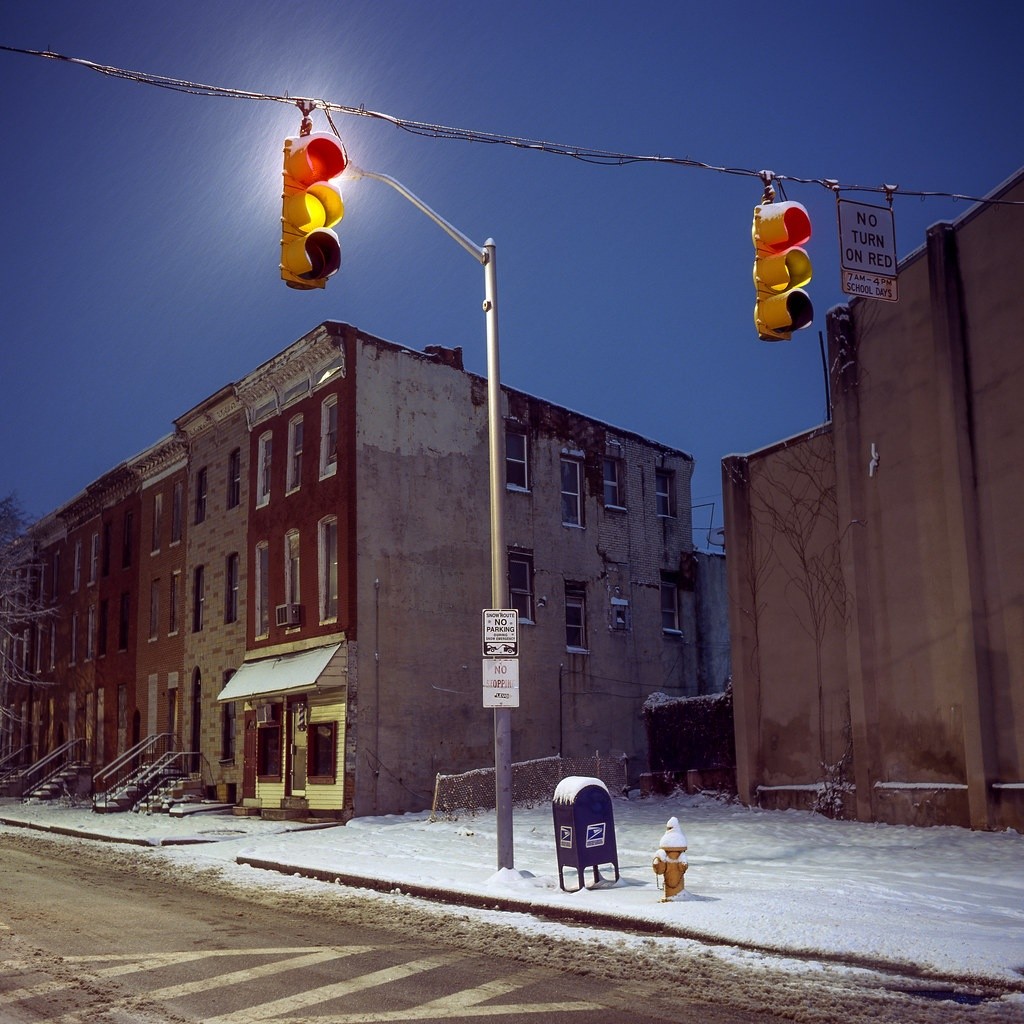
326,164,517,870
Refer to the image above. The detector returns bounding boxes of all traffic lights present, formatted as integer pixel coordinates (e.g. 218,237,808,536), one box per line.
280,134,344,291
754,200,813,342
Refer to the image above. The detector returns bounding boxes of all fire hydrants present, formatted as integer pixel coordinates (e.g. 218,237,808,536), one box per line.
651,819,688,903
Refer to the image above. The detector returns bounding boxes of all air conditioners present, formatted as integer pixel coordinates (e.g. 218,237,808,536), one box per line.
275,603,301,627
